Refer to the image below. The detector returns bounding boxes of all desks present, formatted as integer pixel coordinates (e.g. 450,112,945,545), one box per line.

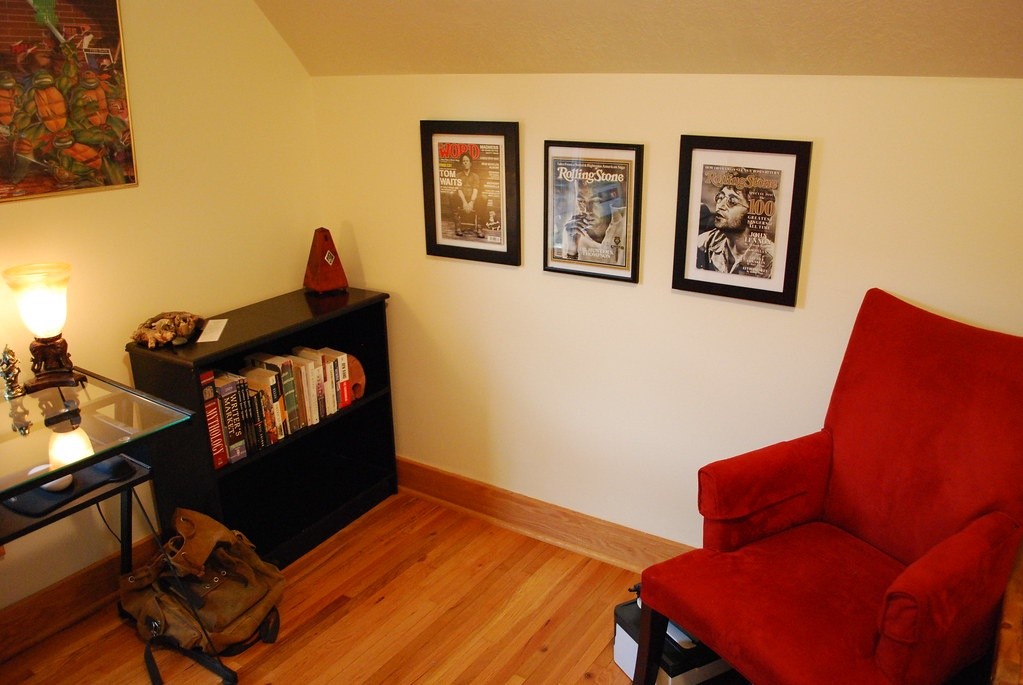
0,364,240,685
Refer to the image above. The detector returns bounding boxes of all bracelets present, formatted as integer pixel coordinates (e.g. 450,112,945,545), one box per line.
567,252,579,260
470,200,475,201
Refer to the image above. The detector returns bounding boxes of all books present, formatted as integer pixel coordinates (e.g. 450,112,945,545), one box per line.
198,345,353,470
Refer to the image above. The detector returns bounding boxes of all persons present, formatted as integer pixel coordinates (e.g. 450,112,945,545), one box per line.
696,166,776,279
560,164,626,267
448,153,488,238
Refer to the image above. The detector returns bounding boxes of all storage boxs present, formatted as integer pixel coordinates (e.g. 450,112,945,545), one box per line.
612,597,736,685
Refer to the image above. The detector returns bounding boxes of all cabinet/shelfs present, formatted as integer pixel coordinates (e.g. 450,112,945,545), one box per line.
124,280,400,572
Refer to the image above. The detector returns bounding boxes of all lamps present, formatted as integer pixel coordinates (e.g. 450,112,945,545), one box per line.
2,259,92,394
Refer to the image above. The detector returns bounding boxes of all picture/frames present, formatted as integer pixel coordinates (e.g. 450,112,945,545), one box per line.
419,119,521,266
0,0,140,203
542,139,645,284
670,135,813,309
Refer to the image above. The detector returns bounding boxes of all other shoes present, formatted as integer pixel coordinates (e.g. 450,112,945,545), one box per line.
477,230,485,238
455,229,463,236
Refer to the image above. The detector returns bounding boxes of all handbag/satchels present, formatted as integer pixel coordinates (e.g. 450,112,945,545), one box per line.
118,506,286,685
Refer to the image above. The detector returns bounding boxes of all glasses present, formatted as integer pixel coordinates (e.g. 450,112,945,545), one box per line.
574,193,619,210
715,191,749,211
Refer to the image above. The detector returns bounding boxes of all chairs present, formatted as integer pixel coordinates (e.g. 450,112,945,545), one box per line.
633,286,1023,685
